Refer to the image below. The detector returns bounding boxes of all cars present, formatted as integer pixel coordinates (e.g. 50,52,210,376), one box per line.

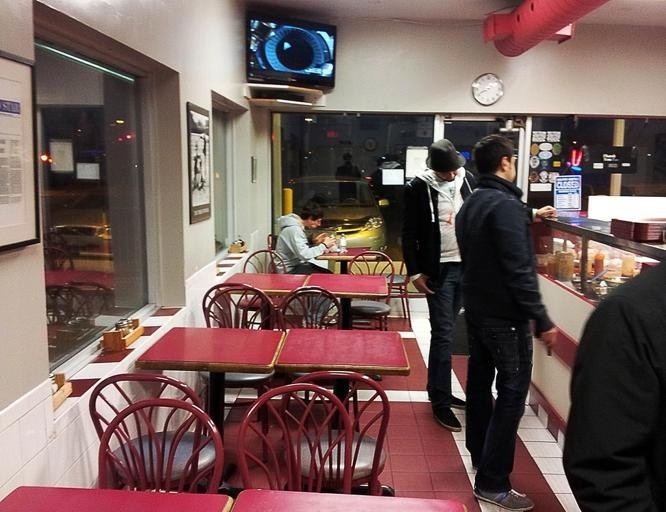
293,177,387,255
53,191,112,248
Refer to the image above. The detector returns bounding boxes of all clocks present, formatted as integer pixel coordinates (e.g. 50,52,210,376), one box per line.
472,73,505,106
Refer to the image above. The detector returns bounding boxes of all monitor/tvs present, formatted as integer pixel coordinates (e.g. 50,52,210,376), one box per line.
382,168,404,185
406,146,430,180
48,139,74,173
581,145,638,173
244,13,337,89
75,161,100,181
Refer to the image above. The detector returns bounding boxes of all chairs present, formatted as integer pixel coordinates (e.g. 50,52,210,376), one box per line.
383,261,411,328
98,399,224,494
236,383,353,494
46,282,115,324
88,374,215,494
275,286,360,433
267,234,278,274
202,283,273,437
348,251,395,331
239,250,286,330
43,232,74,271
280,371,391,496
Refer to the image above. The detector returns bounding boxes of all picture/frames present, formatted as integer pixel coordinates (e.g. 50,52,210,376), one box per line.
185,100,213,225
0,50,40,251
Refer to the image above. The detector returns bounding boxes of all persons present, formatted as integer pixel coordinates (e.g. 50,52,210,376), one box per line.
456,134,557,509
401,138,557,431
272,200,339,326
563,263,666,511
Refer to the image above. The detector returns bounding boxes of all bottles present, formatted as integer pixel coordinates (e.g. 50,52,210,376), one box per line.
120,317,134,330
114,320,127,337
338,233,347,252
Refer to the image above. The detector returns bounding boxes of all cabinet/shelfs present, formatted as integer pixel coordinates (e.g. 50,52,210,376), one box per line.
529,217,666,453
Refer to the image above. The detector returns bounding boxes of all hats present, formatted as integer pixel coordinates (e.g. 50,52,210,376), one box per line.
376,154,390,164
427,139,466,172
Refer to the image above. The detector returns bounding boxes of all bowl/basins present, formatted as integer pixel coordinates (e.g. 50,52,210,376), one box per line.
571,279,592,290
604,277,630,287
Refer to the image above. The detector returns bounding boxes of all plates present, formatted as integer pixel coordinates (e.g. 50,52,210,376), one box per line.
609,216,666,242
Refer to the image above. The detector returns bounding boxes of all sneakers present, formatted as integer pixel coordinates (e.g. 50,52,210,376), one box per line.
473,485,535,511
449,395,468,409
433,408,462,432
